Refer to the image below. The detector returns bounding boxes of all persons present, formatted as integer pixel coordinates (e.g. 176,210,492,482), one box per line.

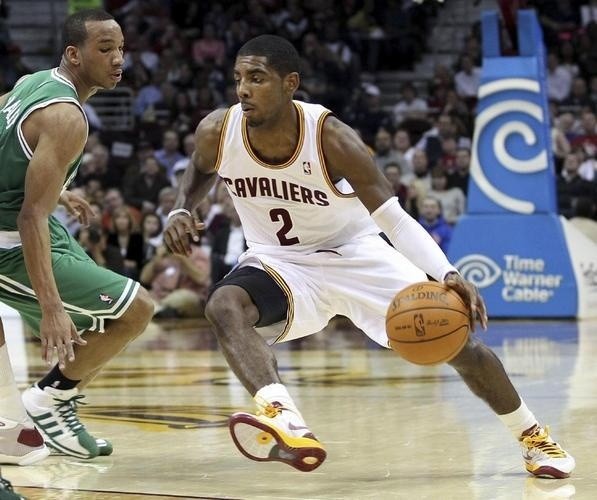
52,1,596,316
0,314,51,466
0,10,157,459
162,32,578,481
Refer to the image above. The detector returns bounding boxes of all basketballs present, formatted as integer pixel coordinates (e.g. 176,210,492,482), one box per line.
386,281,469,367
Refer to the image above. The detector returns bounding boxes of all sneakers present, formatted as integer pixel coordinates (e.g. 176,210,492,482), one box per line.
1,379,113,466
519,424,575,478
229,401,326,472
